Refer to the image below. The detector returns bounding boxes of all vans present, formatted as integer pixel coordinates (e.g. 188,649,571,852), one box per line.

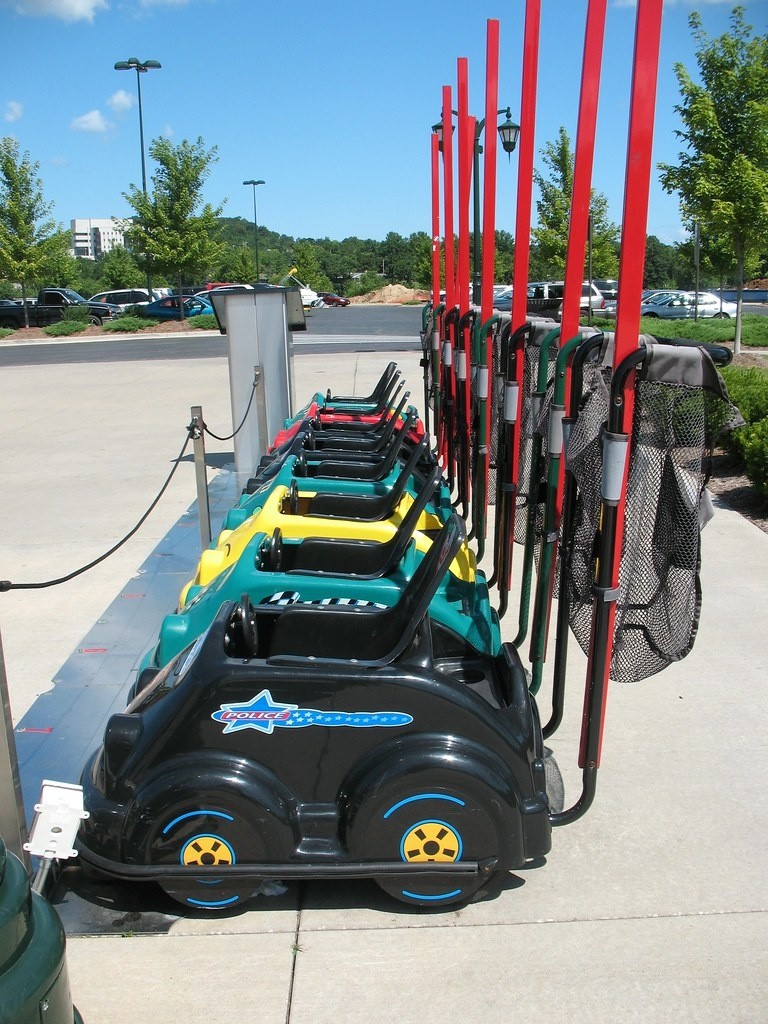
300,288,319,307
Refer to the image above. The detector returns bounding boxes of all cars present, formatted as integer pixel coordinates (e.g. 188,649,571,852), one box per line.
87,282,276,324
317,292,350,307
431,279,738,319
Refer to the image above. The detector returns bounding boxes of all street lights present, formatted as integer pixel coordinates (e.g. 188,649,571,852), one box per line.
243,179,265,287
113,57,163,303
431,106,520,306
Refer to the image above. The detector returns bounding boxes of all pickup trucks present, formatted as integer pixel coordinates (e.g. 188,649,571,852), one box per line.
0,288,123,330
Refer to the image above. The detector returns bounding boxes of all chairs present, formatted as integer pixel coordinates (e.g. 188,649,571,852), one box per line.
265,511,465,668
287,361,443,579
